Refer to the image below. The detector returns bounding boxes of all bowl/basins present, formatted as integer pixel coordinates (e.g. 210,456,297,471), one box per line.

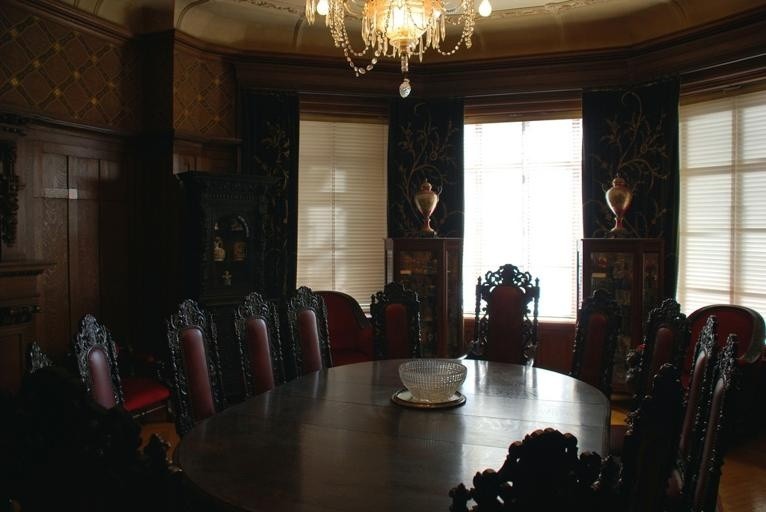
398,359,467,402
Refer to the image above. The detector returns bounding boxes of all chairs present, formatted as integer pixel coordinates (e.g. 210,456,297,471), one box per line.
448,428,637,512
287,287,334,378
463,265,541,367
26,338,55,374
60,333,171,421
165,299,224,433
627,299,688,423
678,333,743,512
624,303,763,442
313,291,373,366
73,313,126,412
566,289,622,398
95,409,198,511
369,282,423,361
622,363,690,512
234,290,287,399
667,315,720,500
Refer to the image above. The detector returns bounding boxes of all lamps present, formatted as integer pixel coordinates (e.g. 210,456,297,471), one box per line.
306,0,493,98
